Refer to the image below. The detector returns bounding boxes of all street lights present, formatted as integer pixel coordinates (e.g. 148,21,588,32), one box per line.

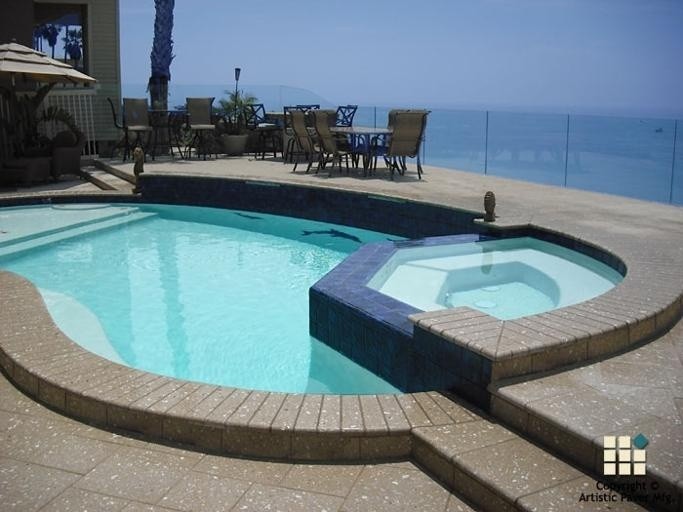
234,66,242,135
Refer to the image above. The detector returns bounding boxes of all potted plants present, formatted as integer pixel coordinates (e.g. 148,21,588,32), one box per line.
212,89,259,156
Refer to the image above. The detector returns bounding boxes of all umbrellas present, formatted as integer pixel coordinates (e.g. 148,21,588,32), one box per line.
0,36,99,155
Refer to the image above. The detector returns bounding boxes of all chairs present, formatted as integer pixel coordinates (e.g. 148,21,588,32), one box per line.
0,131,87,189
106,97,431,182
